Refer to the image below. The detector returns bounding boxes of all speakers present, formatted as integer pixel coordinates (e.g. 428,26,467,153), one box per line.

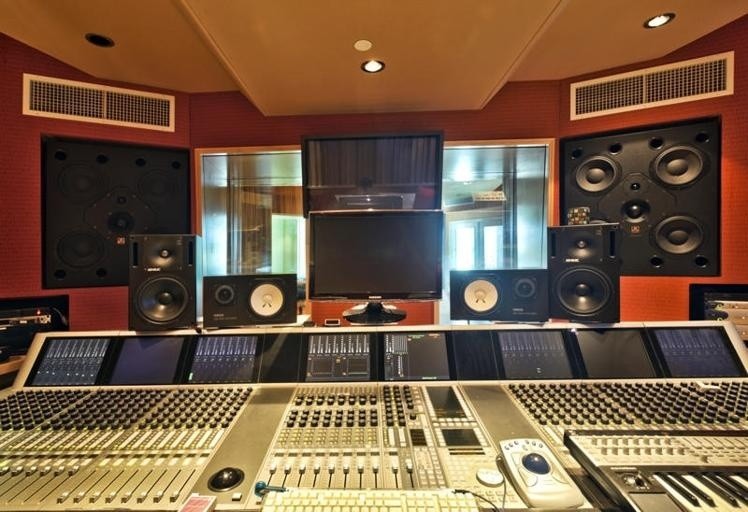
448,267,549,325
547,222,622,323
201,273,297,328
127,233,197,331
557,112,721,278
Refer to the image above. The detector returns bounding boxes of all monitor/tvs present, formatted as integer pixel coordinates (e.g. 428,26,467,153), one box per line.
0,294,70,363
11,320,747,390
688,282,747,347
302,131,445,220
306,208,444,327
